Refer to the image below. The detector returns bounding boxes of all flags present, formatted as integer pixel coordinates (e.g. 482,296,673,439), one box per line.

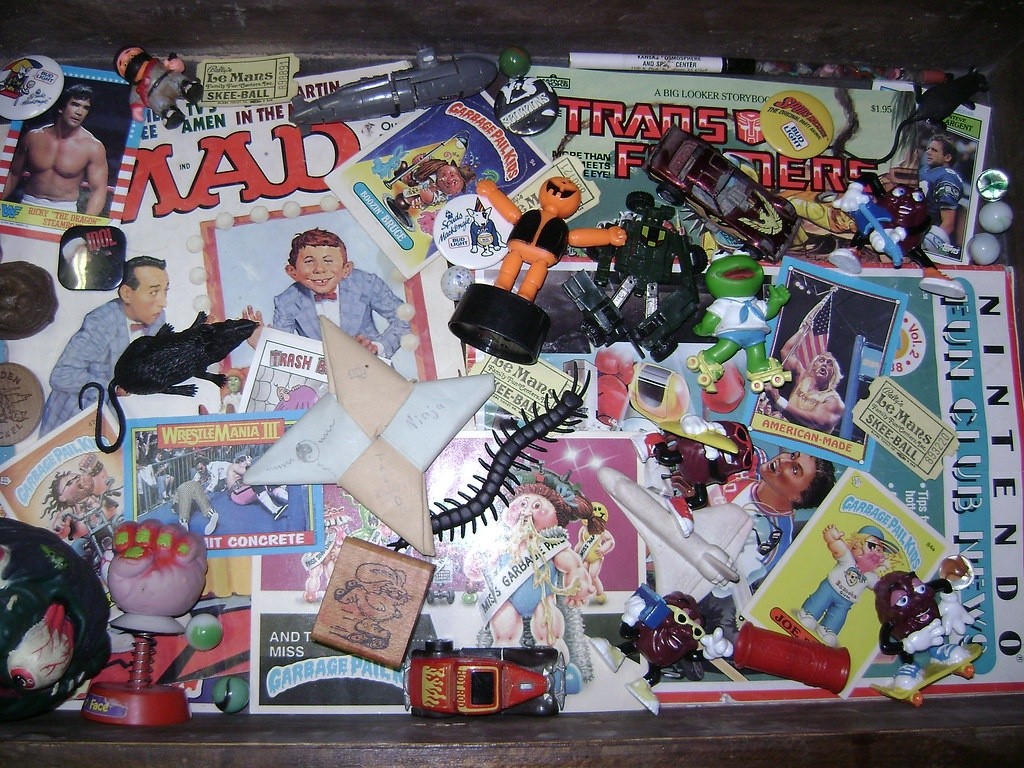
796,291,833,370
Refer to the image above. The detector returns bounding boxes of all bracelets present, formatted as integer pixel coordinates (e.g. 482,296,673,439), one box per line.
774,395,788,413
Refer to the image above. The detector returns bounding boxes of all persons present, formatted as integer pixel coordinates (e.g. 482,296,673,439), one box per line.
763,317,845,435
114,46,204,130
2,84,108,216
916,136,964,255
476,177,627,302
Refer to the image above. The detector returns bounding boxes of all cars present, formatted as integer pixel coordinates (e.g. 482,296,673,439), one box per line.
407,637,570,719
640,120,804,260
